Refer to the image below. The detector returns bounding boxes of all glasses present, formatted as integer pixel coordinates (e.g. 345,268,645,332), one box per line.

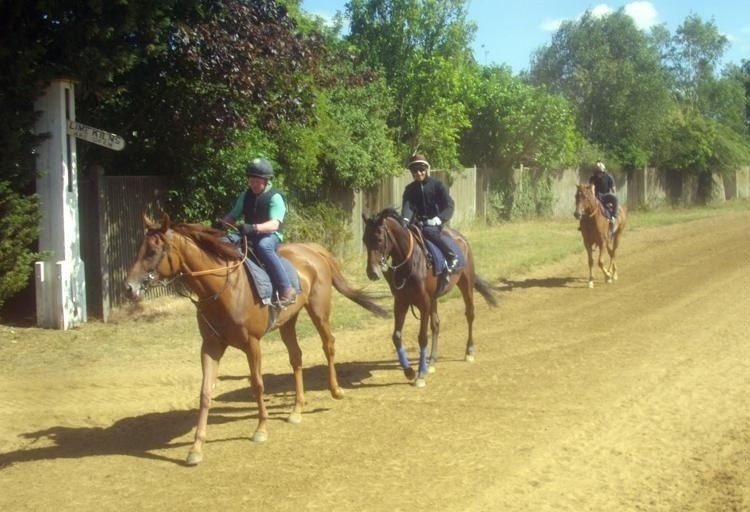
411,164,427,171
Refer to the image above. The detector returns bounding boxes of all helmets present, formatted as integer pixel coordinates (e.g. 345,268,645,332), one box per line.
246,158,273,178
408,155,430,168
594,161,605,173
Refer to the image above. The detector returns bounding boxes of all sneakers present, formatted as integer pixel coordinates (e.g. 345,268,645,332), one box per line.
274,288,296,304
610,216,617,224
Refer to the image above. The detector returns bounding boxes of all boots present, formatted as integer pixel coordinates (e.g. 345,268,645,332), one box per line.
436,235,465,272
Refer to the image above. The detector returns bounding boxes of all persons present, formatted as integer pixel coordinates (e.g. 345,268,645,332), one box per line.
212,158,297,305
401,155,461,269
590,160,618,224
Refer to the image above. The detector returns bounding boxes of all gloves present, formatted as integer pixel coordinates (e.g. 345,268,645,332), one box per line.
238,223,254,235
427,219,436,225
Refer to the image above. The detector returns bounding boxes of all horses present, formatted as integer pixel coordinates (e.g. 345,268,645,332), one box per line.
573,181,628,289
123,212,391,469
360,204,501,389
